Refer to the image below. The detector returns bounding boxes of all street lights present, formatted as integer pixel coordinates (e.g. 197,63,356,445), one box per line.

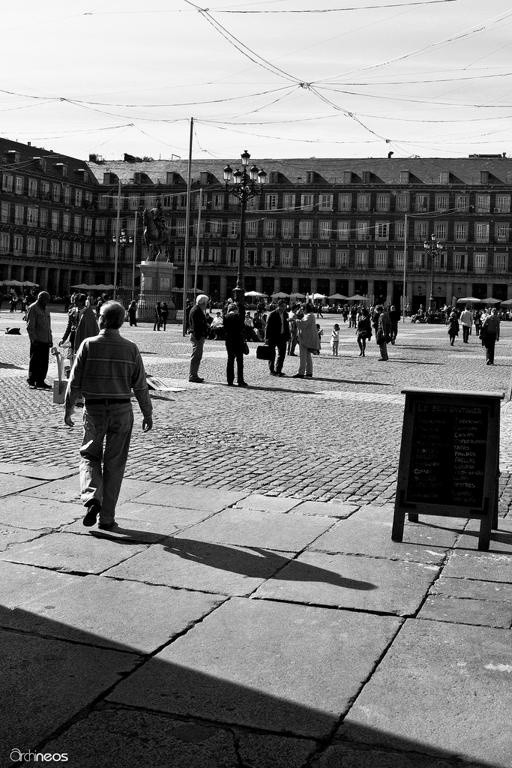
224,149,267,302
423,234,444,320
112,228,134,310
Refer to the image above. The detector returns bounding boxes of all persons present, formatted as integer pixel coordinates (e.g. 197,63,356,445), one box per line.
64,299,153,530
9,289,512,389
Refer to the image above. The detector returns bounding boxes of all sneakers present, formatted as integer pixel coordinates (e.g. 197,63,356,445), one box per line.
293,374,304,378
378,358,387,361
238,381,248,386
189,377,204,382
227,380,233,385
306,373,312,377
83,503,99,526
98,522,117,530
289,352,297,356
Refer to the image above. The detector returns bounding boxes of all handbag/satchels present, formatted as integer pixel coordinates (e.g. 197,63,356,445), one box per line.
257,346,275,360
243,342,249,354
